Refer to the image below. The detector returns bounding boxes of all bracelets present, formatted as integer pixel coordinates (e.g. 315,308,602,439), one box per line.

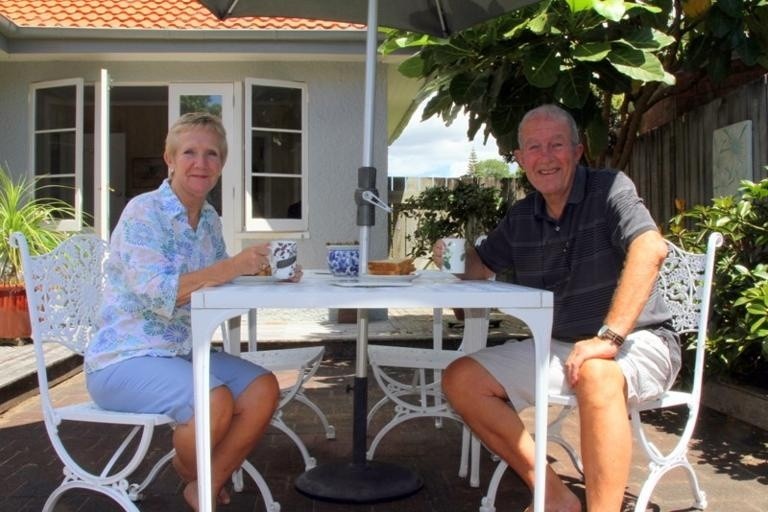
597,324,625,345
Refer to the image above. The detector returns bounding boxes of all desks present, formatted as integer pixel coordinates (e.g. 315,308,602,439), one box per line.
184,261,553,502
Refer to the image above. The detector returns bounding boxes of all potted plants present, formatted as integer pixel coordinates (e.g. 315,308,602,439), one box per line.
0,159,112,343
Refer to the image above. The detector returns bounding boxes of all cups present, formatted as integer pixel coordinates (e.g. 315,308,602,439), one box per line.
264,239,297,280
441,237,467,274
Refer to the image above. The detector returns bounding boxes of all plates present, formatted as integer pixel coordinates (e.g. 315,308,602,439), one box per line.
366,273,419,282
231,275,276,284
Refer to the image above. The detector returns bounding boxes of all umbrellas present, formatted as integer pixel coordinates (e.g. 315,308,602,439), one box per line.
201,0,553,496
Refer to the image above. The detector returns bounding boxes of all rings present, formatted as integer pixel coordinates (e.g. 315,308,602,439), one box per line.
259,263,267,270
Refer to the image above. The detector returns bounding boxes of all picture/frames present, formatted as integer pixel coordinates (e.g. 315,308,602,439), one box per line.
129,156,168,189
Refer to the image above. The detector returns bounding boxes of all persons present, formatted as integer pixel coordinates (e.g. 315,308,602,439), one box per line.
431,103,685,512
83,111,303,512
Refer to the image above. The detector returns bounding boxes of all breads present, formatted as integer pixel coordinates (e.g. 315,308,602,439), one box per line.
368,258,416,275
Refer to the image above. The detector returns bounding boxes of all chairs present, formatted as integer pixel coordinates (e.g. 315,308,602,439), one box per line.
12,230,293,512
360,281,500,464
217,317,339,494
477,232,726,512
